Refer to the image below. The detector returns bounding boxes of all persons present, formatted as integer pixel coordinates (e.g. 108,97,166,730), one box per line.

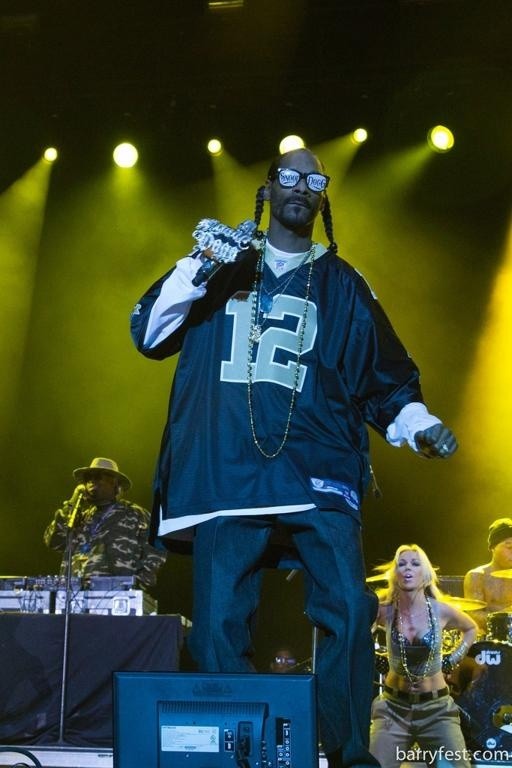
463,518,512,643
131,148,459,767
42,457,166,602
365,545,478,767
269,641,295,674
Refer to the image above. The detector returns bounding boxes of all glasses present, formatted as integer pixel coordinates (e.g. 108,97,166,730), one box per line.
269,168,332,191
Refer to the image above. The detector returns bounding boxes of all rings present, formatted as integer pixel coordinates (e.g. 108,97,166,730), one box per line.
442,443,448,452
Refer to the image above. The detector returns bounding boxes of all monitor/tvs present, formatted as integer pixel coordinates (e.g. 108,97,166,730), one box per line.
110,671,319,768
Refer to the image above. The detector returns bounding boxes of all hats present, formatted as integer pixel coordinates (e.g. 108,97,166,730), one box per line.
487,517,512,552
72,457,131,493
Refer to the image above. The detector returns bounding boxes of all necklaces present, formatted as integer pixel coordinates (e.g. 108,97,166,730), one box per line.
80,501,117,554
392,596,438,684
248,227,315,458
250,248,311,343
259,269,292,312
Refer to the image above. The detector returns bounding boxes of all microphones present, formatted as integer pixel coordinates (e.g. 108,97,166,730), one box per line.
67,484,86,529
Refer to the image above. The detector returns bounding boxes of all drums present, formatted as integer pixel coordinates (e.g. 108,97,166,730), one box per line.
444,598,489,632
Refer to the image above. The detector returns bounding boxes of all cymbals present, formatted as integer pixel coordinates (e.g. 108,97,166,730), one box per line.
491,569,512,578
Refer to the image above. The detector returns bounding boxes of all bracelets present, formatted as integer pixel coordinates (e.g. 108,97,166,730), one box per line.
447,640,468,666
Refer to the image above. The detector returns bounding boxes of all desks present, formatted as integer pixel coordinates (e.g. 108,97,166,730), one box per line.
0,611,192,751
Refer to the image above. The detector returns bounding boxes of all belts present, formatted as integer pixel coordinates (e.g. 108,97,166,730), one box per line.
381,682,449,702
